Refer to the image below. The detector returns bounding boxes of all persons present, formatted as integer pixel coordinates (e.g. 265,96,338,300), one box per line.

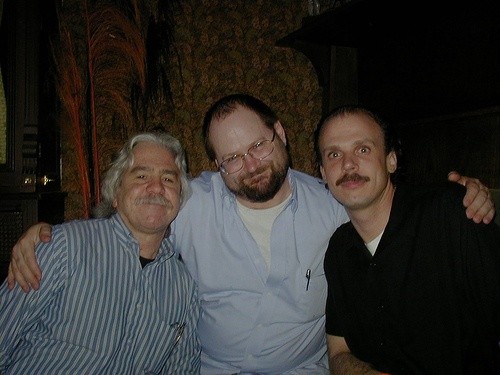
314,102,500,375
8,95,495,375
0,134,201,375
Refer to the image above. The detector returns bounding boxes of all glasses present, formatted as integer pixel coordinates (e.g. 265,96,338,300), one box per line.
216,129,276,174
